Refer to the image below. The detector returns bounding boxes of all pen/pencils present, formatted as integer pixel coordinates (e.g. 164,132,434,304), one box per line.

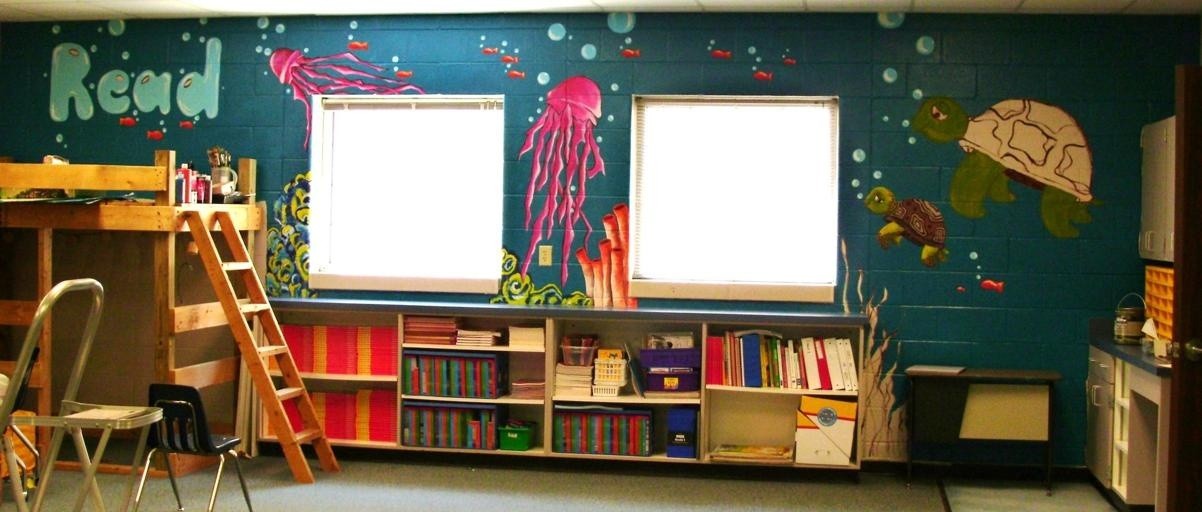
207,146,232,168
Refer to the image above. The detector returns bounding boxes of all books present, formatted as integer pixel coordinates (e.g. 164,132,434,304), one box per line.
402,353,545,400
552,405,654,457
400,399,510,450
267,388,397,442
707,441,795,465
403,316,545,351
268,322,398,377
705,326,860,392
554,331,695,398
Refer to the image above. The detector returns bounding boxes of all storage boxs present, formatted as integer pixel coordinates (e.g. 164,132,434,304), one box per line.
559,342,599,366
647,370,700,392
639,344,701,369
496,421,537,451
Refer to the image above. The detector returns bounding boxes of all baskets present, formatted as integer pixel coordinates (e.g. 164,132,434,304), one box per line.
592,360,626,397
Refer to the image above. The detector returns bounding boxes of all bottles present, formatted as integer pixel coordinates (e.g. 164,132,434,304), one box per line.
176,164,191,203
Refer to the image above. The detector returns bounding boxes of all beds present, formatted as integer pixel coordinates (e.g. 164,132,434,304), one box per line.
0,151,264,479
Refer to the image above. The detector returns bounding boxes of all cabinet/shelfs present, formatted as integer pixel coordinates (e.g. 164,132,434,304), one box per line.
1084,115,1177,512
258,297,869,485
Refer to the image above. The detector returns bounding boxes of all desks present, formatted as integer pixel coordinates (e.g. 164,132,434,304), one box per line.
904,364,1062,497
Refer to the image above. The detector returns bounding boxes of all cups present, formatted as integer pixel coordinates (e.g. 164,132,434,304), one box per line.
211,166,239,193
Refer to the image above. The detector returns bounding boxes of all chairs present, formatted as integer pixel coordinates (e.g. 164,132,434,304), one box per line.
1,346,43,503
132,383,253,512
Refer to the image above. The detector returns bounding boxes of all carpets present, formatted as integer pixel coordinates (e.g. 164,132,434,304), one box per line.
0,437,951,512
